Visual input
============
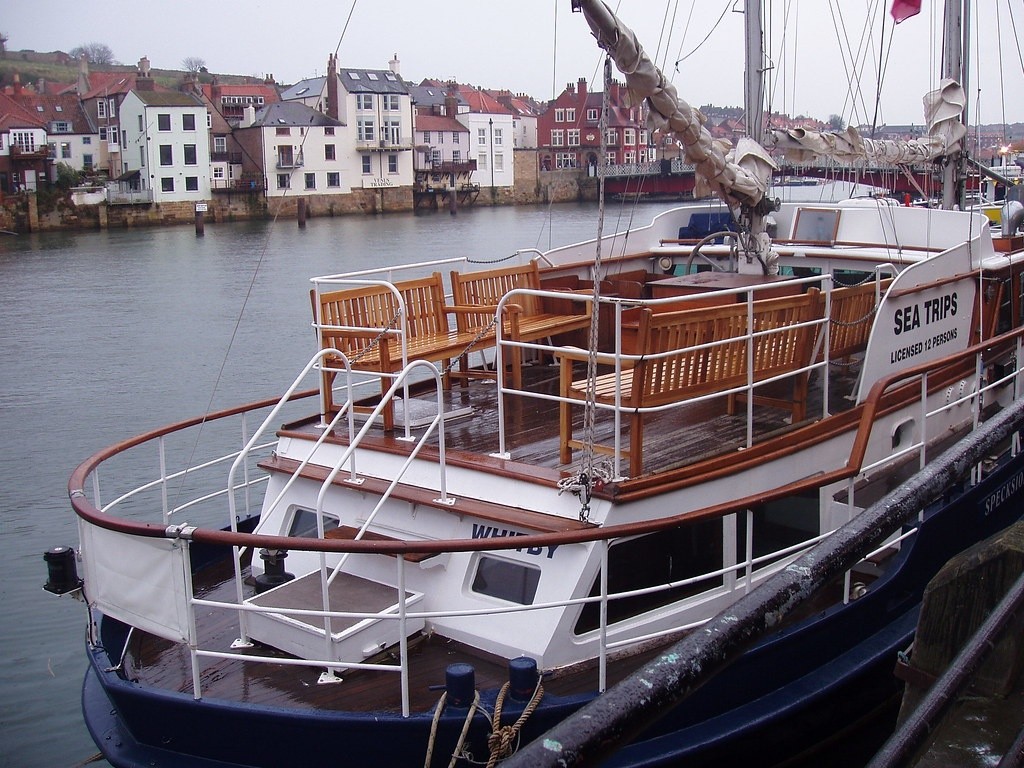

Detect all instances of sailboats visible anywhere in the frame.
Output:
[38,0,1024,768]
